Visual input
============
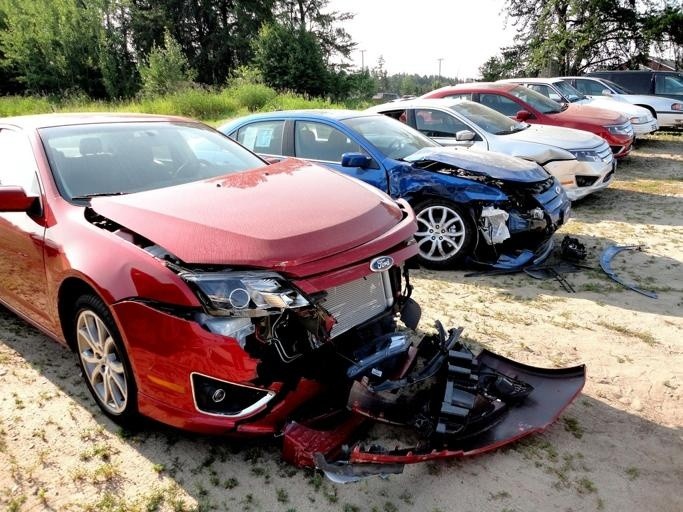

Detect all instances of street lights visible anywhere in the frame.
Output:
[355,48,368,86]
[434,56,446,88]
